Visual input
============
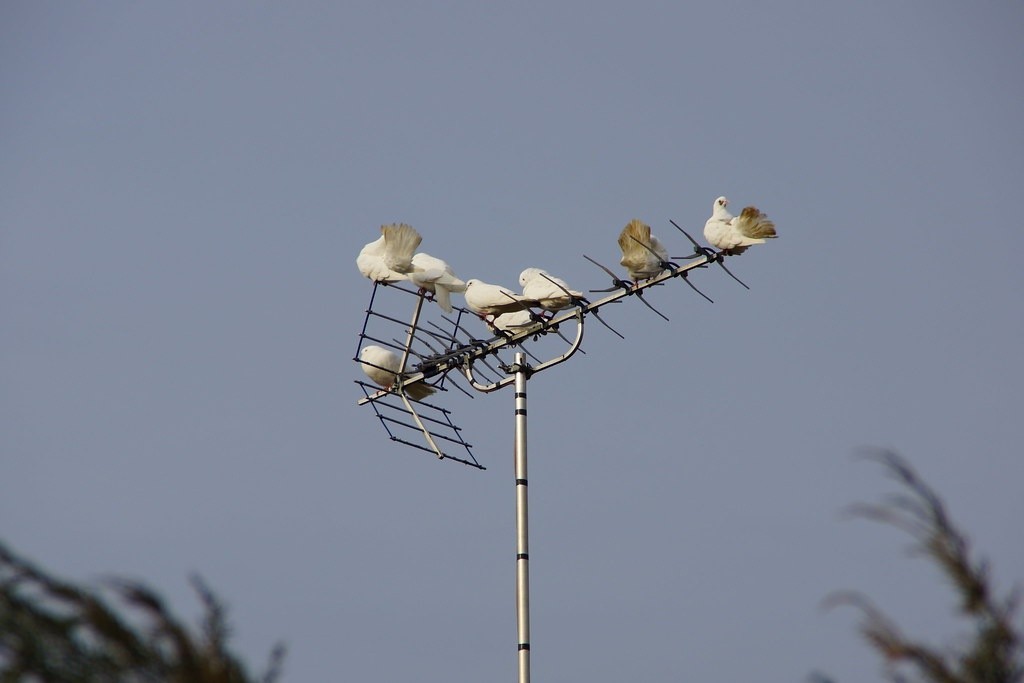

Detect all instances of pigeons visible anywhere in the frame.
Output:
[361,345,436,400]
[355,195,776,334]
